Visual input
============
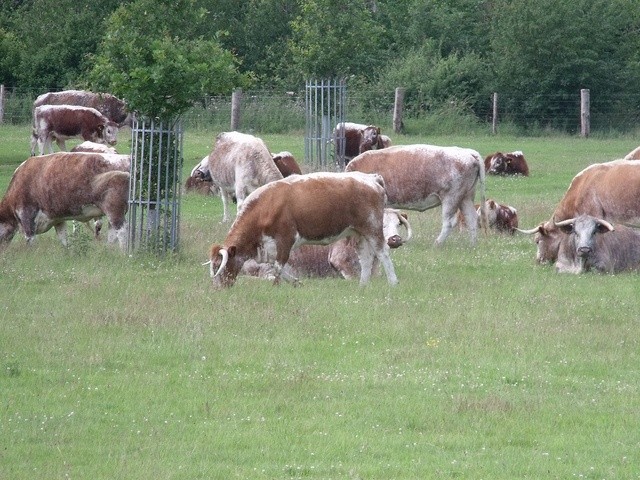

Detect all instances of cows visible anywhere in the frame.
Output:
[70,139,121,240]
[484,150,529,177]
[198,173,401,298]
[511,158,640,266]
[0,151,141,252]
[345,143,491,249]
[185,150,302,205]
[236,207,411,284]
[32,90,143,154]
[448,197,519,237]
[333,122,381,171]
[552,214,640,277]
[208,131,285,226]
[30,104,120,157]
